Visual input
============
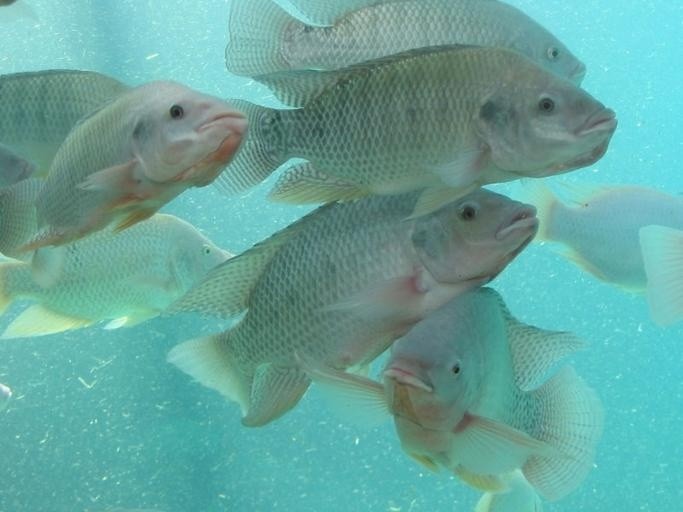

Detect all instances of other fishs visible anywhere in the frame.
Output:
[0,0,681,510]
